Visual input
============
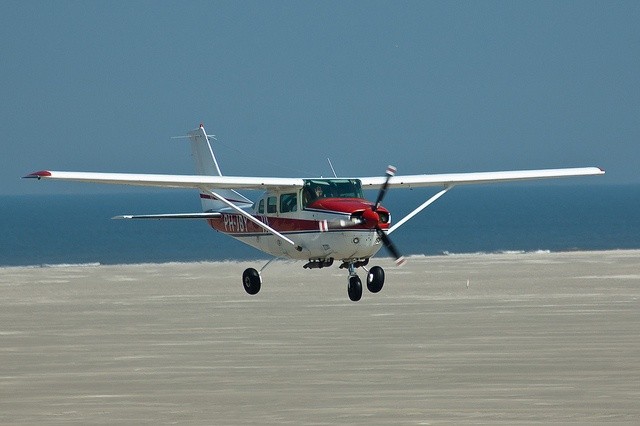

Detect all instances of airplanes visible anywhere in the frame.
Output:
[19,122,605,303]
